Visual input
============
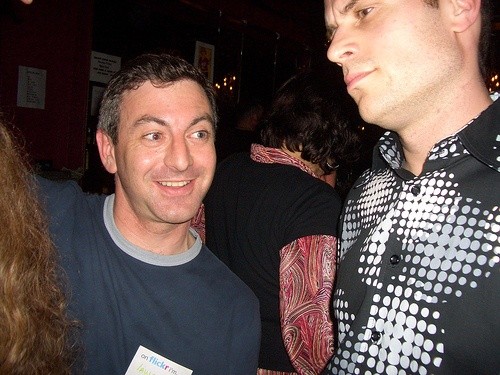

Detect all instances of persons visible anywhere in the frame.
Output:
[0,125,81,375]
[320,0,500,375]
[333,115,368,183]
[28,54,261,375]
[224,101,261,152]
[192,74,343,375]
